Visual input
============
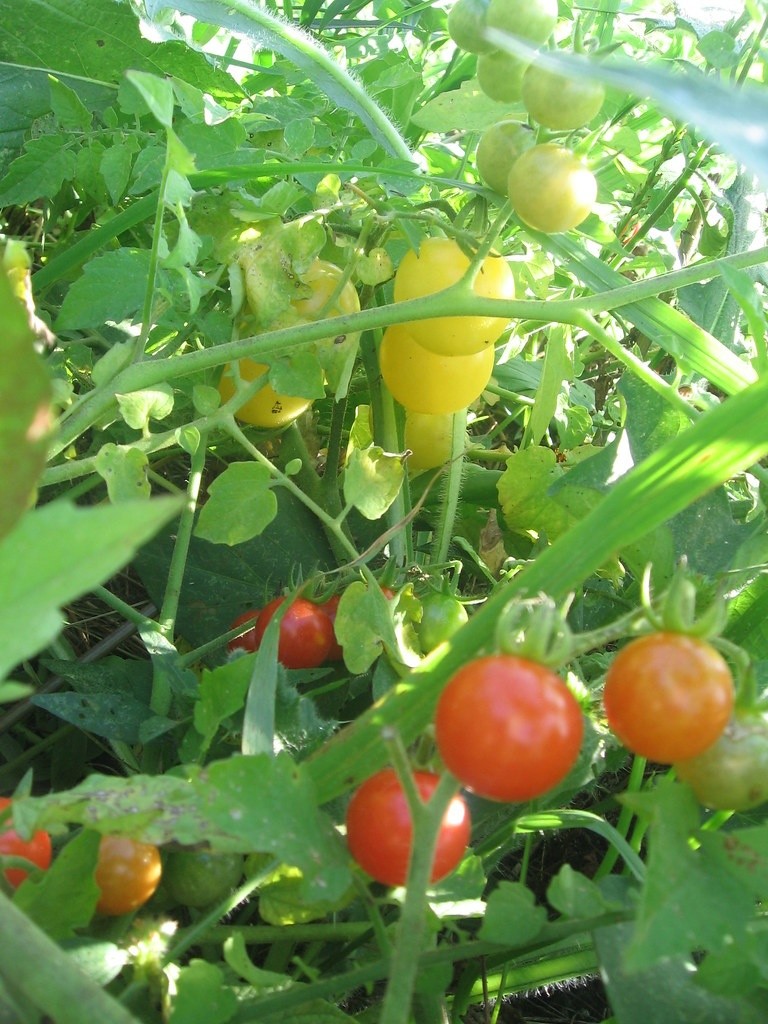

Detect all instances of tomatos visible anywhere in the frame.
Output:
[0,0,767,915]
